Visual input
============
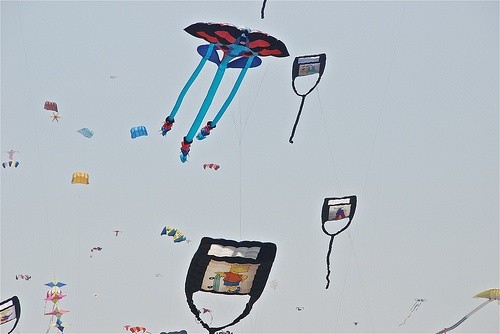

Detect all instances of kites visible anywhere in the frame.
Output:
[0,224,191,334]
[160,22,289,164]
[436,289,500,334]
[322,195,357,289]
[398,298,429,326]
[0,95,149,185]
[185,238,277,334]
[203,163,220,171]
[291,53,326,143]
